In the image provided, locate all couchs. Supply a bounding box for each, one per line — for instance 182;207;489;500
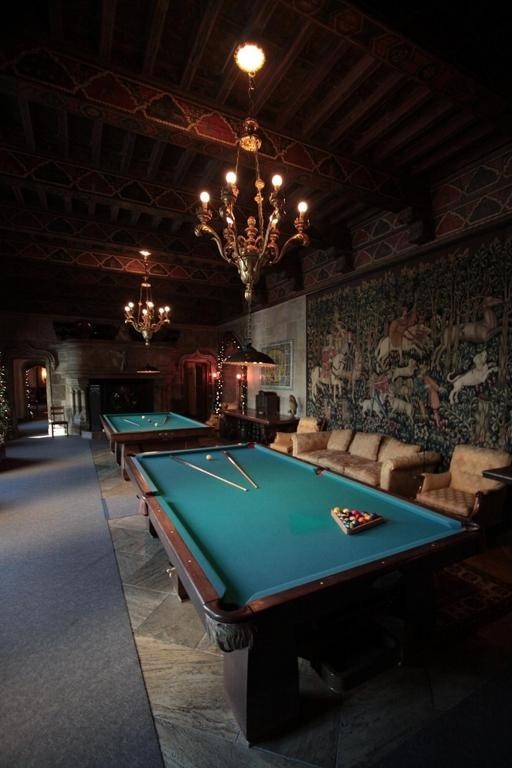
292;431;443;496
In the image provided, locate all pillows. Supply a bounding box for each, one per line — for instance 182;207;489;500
350;431;381;460
378;435;421;463
327;429;355;452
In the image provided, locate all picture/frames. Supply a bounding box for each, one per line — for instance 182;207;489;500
259;340;293;390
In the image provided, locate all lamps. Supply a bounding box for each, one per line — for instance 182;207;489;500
236;373;244;380
195;41;311;301
41;366;46;383
123;251;171;347
223;301;277;367
212;372;220;379
136;363;161;375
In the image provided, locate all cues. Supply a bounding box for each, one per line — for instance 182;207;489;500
175;456;247;492
123;419;140;427
163;415;168;424
223;449;259;490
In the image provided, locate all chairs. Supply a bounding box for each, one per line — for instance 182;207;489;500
413;445;512;551
270;416;325;454
206;403;239;440
50;406;68;438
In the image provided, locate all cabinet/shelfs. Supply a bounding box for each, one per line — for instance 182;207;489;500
224;408;300;446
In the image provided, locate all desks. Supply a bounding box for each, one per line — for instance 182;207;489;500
124;442;483;748
100;411;217;480
482;465;512;485
